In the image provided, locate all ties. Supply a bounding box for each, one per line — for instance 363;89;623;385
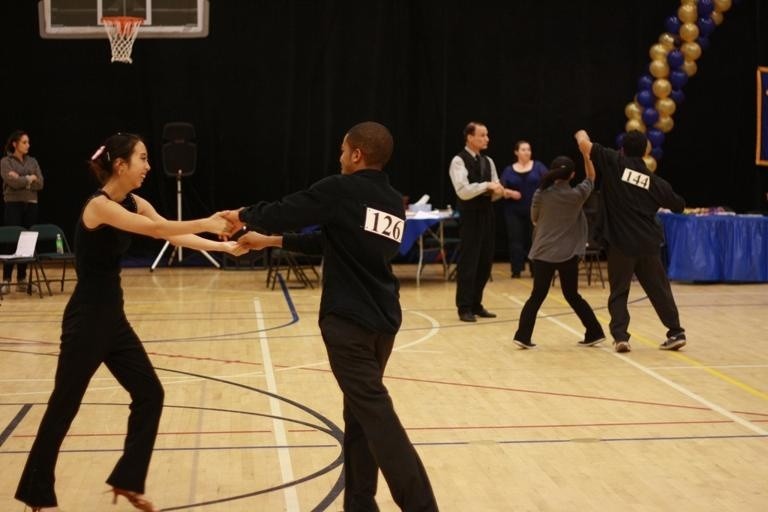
476;154;485;178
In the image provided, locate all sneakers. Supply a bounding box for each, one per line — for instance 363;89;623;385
660;335;686;350
578;337;606;346
1;281;10;295
16;279;37;293
513;340;537;349
616;341;632;352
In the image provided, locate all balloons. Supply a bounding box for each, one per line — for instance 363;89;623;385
616;1;733;173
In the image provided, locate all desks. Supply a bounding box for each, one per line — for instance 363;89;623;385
398;210;459;288
660;213;767;282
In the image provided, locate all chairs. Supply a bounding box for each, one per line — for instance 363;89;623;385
552;233;606;290
32;223;79;298
0;226;43;297
265;236;321;289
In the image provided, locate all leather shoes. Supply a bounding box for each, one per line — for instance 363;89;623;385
461;311;475;322
477;310;496;317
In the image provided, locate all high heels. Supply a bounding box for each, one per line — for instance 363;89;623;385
113;487;160;512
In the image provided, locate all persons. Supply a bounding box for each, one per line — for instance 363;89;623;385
575;127;687;351
512;139;607;350
0;132;45;295
499;141;552;280
218;120;441;512
13;132;252;511
448;120;512;322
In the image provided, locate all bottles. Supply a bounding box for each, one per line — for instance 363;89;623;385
55;233;64;255
